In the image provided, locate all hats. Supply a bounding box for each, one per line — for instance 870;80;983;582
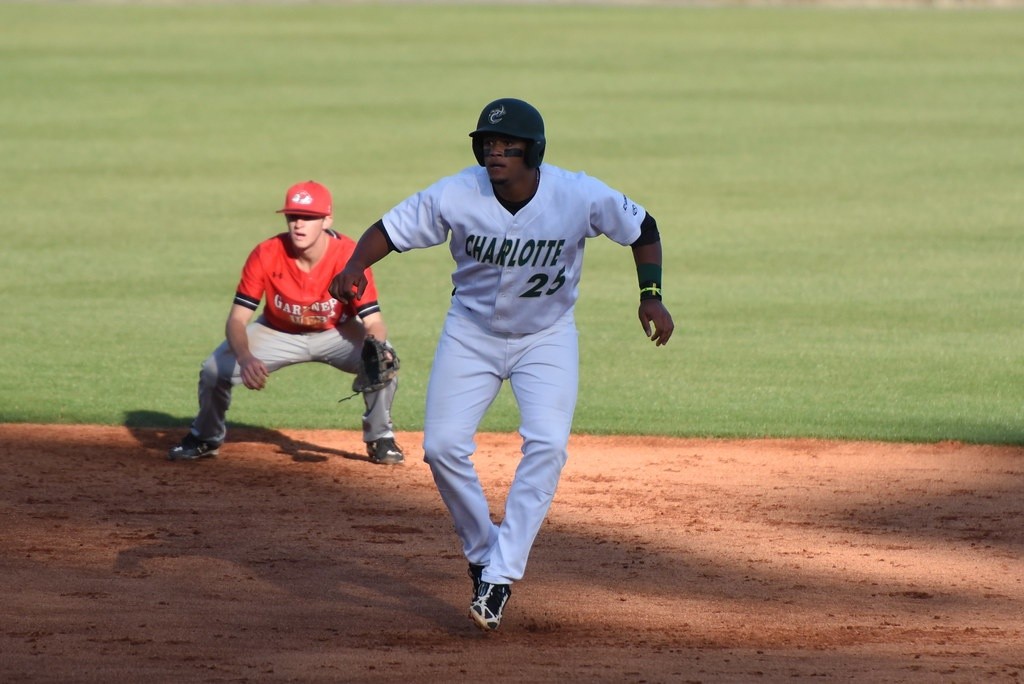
276;180;331;216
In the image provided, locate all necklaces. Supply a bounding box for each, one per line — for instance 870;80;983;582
535;169;540;184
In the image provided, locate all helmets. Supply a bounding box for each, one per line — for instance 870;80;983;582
469;98;546;167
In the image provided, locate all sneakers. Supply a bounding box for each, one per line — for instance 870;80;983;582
366;438;404;463
470;582;512;631
468;562;488;633
170;432;220;459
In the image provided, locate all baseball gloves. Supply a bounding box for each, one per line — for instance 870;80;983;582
362;332;400;392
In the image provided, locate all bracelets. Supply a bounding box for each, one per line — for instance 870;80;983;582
640;283;662;297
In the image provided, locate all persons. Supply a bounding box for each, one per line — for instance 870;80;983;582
167;177;407;465
328;97;675;630
